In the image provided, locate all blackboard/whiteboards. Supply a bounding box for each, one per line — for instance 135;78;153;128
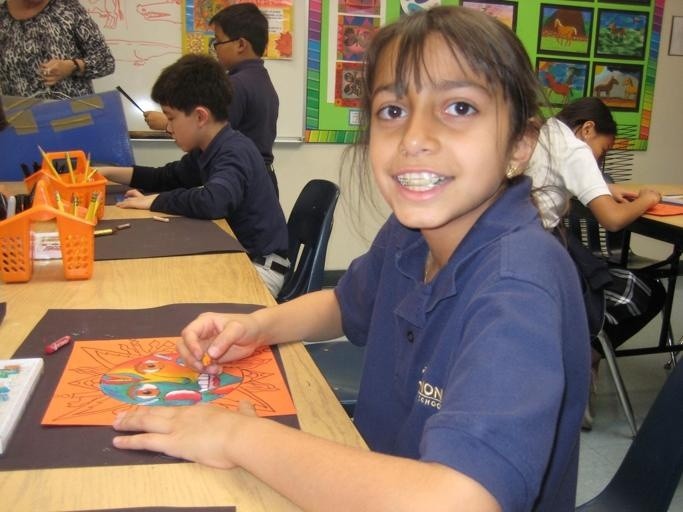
78;0;308;144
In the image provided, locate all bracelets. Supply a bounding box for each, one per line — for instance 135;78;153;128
72;58;80;72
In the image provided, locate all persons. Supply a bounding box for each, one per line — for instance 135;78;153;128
0;0;116;101
143;3;280;201
520;94;665;432
85;53;290;300
110;7;591;510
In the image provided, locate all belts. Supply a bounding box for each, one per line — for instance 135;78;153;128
250;255;288;274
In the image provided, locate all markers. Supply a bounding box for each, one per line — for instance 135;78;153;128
45;336;70;354
95;228;116;236
118;224;131;231
153;216;170;223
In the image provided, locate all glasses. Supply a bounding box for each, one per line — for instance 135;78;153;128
213;38;240;52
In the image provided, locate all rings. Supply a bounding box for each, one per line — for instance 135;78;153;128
40;70;49;75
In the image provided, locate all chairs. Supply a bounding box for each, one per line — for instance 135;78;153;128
566;172;678;368
575;348;683;512
276;178;339;302
307;341;366;418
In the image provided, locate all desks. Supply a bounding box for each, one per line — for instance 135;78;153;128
610;181;683;359
0;184;400;512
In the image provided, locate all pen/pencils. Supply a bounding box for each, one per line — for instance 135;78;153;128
0;192;31;220
55;189;100;222
37;144;98;184
201;351;212;368
21;159;41;178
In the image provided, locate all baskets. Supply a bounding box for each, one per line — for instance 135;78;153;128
26;151;108;219
1;181;96;283
